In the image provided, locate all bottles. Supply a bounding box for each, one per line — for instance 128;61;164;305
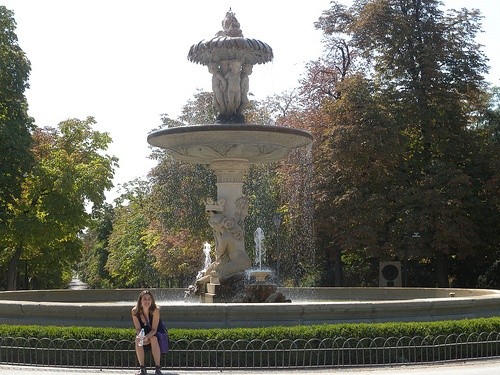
138;328;145;346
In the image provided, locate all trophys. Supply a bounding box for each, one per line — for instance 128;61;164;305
208;214;252;283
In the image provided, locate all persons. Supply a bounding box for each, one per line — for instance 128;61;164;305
220;60;241;114
208;63;227;114
131;290;168;375
240;63;253;114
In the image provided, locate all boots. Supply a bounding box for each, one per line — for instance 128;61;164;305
154;365;163;375
135;365;147;375
